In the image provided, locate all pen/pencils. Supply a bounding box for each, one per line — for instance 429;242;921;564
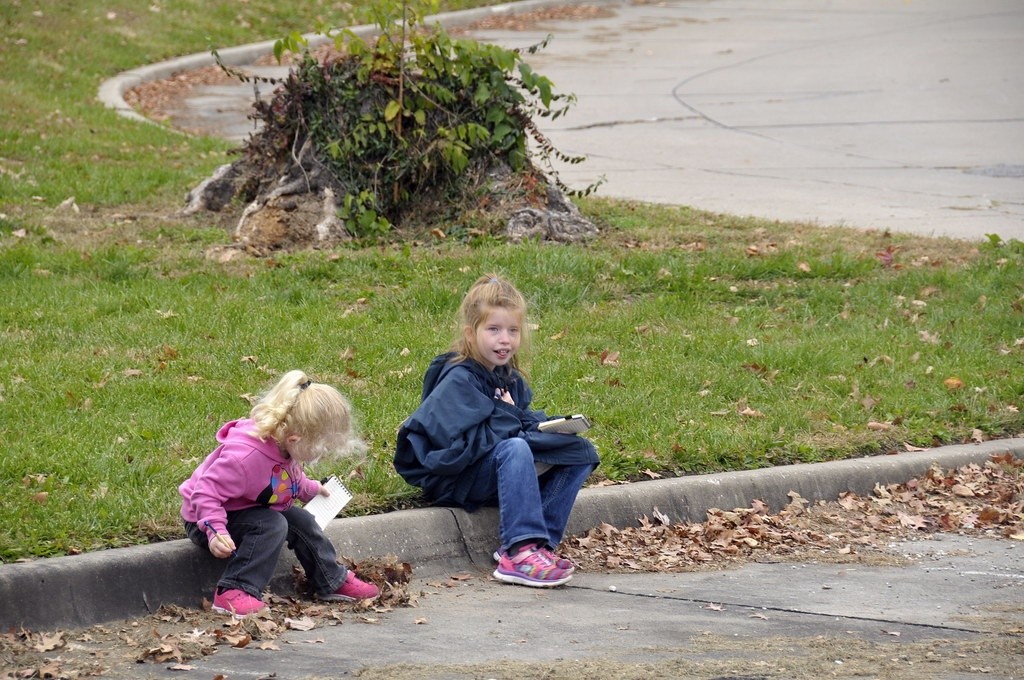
495;393;503;400
204;520;239;558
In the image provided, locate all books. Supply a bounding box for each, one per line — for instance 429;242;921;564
300;473;354;532
537;413;592;434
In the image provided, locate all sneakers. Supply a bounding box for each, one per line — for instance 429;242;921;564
211;587;271;619
493;544;572;587
314;570;380;602
493;545;574;574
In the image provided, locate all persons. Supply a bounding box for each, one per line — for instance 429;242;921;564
392;272;601;588
178;370;380;621
